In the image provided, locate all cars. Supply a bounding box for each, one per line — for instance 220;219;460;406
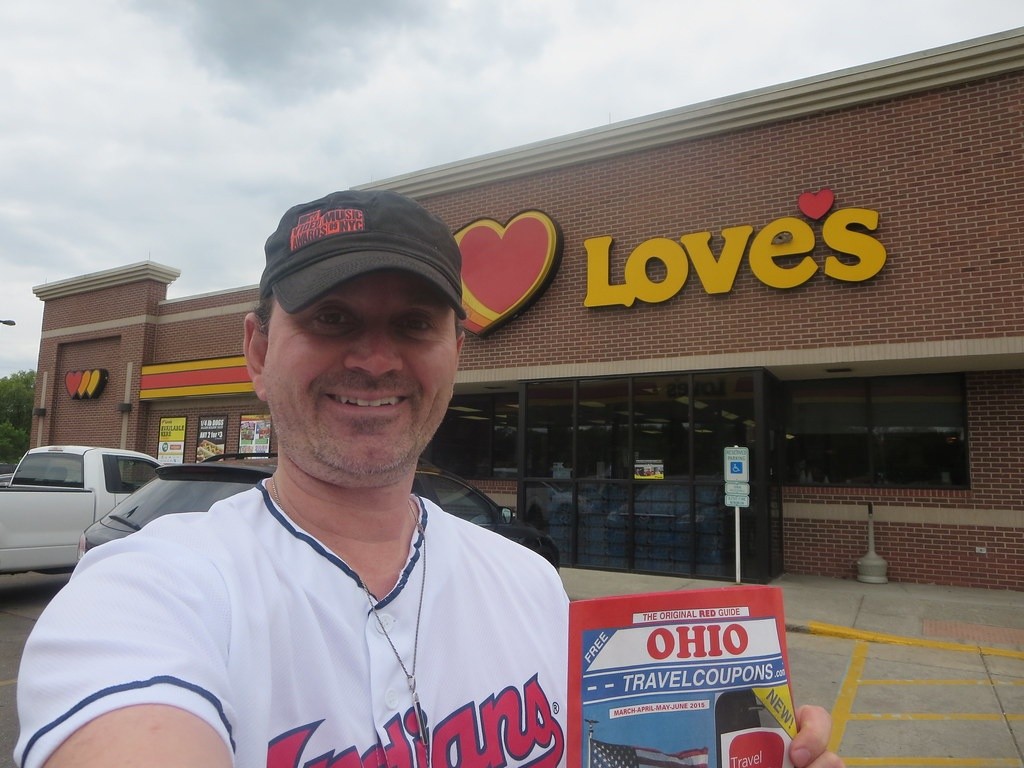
604;477;760;565
493;468;592;527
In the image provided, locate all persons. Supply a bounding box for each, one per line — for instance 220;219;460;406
15;191;845;768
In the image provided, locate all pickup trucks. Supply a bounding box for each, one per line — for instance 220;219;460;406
0;446;165;575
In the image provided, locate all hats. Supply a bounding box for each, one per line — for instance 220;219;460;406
258;189;467;320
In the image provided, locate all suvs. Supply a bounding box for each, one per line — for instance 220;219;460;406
78;453;559;573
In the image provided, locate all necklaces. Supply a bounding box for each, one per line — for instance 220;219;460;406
267;475;431;745
